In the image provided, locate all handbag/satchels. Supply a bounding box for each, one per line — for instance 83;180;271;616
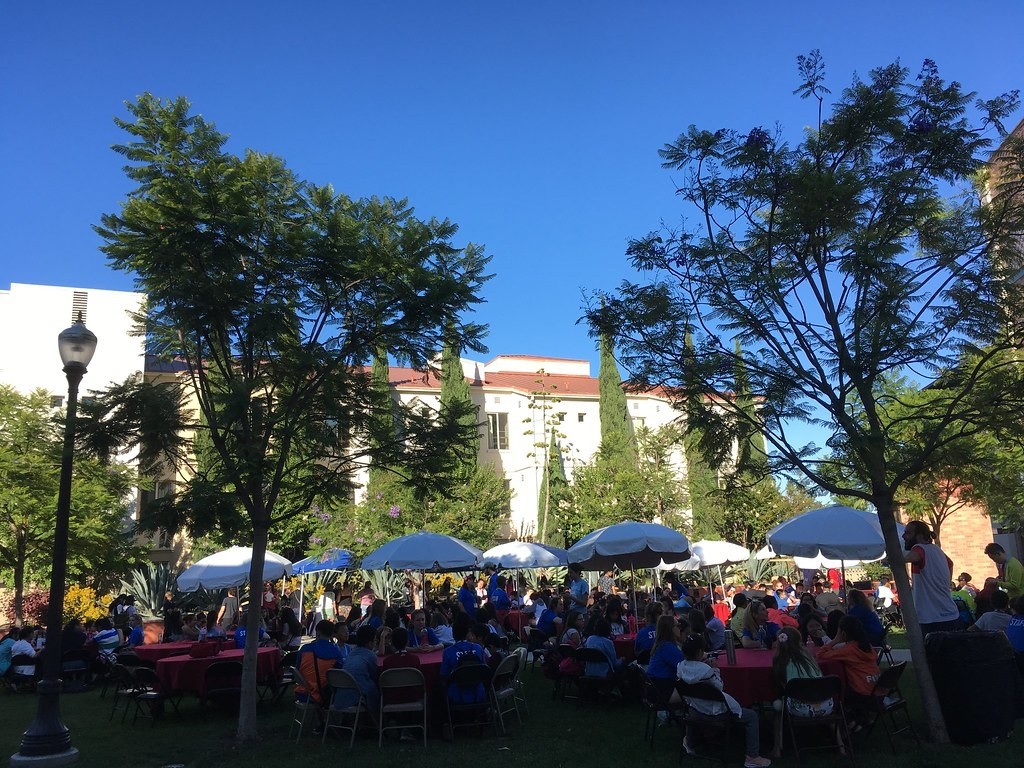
320;685;334;710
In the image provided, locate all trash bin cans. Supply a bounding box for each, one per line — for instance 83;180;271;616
924;630;1018;747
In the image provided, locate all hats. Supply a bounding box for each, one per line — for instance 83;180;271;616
464;576;473;582
482;562;495;570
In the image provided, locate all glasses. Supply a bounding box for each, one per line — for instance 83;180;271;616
577;618;585;622
673;623;681;629
751;600;758;607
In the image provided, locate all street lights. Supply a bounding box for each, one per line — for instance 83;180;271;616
6;306;99;768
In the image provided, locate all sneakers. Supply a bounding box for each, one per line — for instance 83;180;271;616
744;755;771;768
683;736;695;756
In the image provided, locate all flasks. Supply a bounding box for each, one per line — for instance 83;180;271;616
724;630;737;665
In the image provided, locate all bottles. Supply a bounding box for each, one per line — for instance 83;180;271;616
198;627;207;641
629;617;636;635
758;625;766;648
421;627;428;647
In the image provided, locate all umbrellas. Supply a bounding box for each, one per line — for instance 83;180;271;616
754;502;912;616
176;521;750;646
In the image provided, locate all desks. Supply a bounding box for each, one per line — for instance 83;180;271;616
152;646;283;693
710;604;731;628
611;635;636;659
703;646;846;701
133;639;236;662
509;610;528;641
377;649;444;673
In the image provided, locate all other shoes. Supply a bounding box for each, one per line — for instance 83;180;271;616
841;715;874;739
11;684;21;694
443;727;451;742
400;729;416;741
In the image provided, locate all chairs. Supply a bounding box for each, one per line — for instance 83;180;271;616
10;598;975;766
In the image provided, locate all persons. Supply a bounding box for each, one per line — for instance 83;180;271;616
881;521;1024;655
291;562;898;768
0;594;144;694
163;581;309;651
